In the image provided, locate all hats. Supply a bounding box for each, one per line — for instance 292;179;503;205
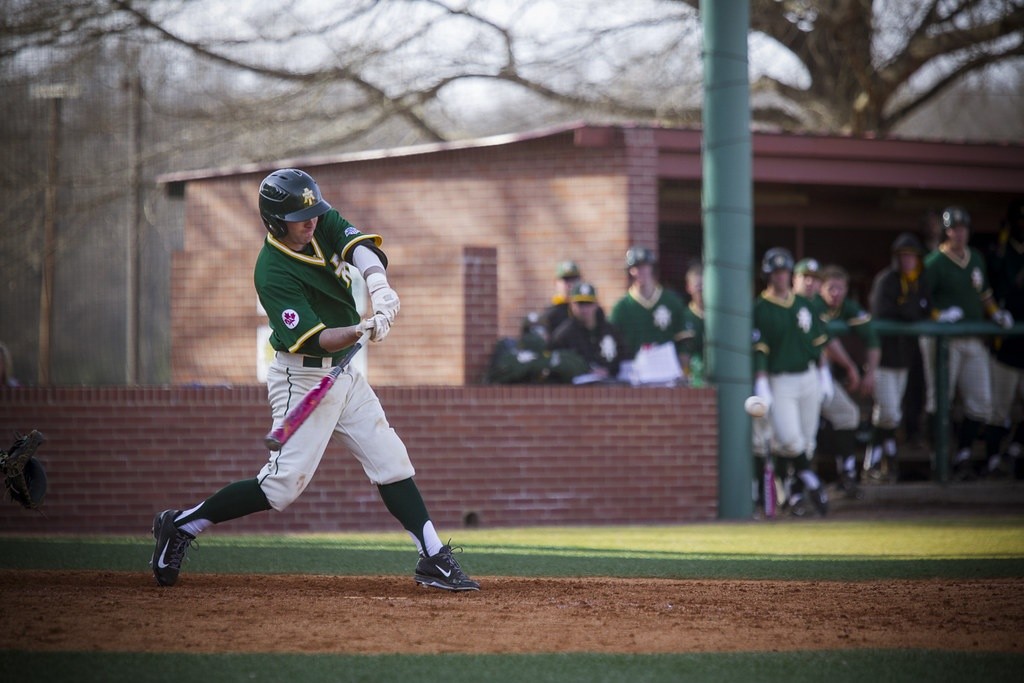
556;262;580;277
762;248;794;273
626;246;653;269
794;257;822;277
523;314;551;331
894;233;922;255
571;283;597;302
822;264;847;277
943;205;971;226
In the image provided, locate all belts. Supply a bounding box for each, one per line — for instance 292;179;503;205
302;356;343;367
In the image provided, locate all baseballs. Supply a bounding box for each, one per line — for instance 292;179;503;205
743;395;769;418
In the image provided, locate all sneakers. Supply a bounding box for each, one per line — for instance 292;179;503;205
149;508;199;586
415;538;482;590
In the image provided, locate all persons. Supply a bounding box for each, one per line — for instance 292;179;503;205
488;207;1024;518
150;169;481;591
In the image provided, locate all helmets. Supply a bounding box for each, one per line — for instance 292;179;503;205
258;169;331;238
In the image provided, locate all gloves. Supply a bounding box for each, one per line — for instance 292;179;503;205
993;308;1014;328
371;287;401;327
356;314;390;342
937;306;964;323
820;369;834;404
756;377;772;417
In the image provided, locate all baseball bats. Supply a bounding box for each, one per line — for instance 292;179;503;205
260;329;374;452
